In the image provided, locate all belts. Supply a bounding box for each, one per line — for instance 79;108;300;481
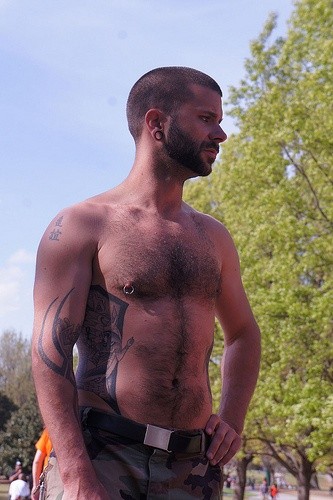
76;406;213;456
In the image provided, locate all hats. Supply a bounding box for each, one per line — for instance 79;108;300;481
15;461;21;465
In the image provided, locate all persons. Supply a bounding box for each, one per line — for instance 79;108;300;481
30;66;262;500
221;471;279;500
7;427;56;499
